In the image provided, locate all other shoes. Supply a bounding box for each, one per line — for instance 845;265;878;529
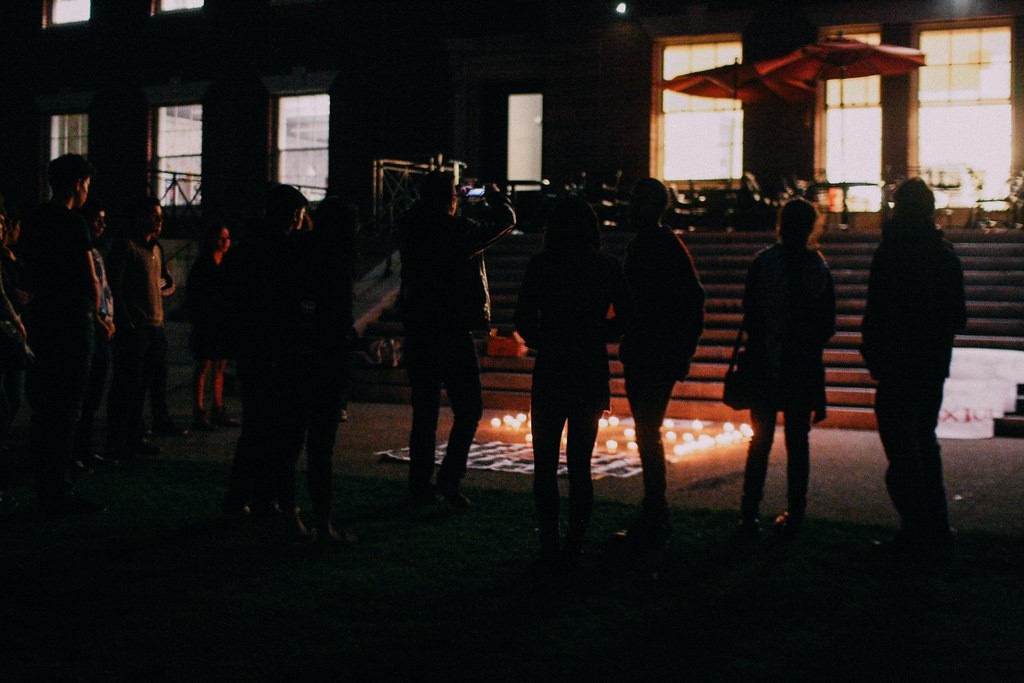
286;507;337;541
405;484;471;509
79;415;189;464
30;490;107;517
876;530;952;564
614;497;670;552
733;524;800;556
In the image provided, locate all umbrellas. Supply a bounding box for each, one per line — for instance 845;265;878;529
654;33;926;181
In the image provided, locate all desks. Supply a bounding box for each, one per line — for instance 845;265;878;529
812;182;877;226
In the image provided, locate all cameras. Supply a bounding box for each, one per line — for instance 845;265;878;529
467;186;487;196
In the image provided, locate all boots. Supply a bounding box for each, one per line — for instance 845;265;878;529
191;399;241;432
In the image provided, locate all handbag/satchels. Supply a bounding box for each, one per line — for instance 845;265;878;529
723;361;757;408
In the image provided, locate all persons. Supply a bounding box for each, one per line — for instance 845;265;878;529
0;154;189;515
859;177;967;560
727;173;760;231
613;177;707;545
185;185;396;546
398;173;515;512
516;196;622;560
736;199;836;537
